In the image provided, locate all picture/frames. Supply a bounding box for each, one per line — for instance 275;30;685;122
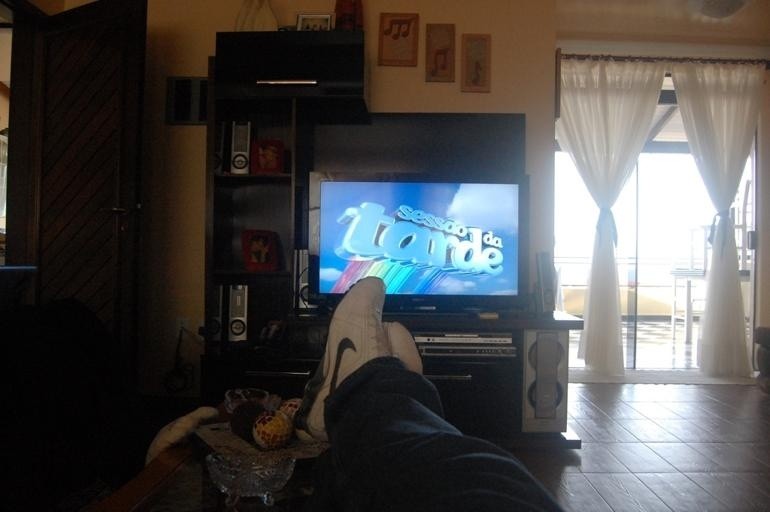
296;14;333;32
377;11;455;81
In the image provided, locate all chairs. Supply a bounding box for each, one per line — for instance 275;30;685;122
670;224;749;344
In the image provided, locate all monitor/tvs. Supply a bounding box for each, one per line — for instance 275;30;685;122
308;171;530;316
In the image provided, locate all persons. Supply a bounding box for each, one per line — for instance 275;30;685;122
293;276;566;512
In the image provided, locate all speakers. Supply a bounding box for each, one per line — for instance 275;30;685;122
298;250;308;310
230;120;251;175
228;284;248;342
521;329;569;433
535;251;555;312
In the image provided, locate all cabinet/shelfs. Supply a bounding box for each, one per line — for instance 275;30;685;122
202;30;585;453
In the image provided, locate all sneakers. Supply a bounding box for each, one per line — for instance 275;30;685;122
294;276;424;445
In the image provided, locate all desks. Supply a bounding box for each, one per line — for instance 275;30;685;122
82;394;316;511
